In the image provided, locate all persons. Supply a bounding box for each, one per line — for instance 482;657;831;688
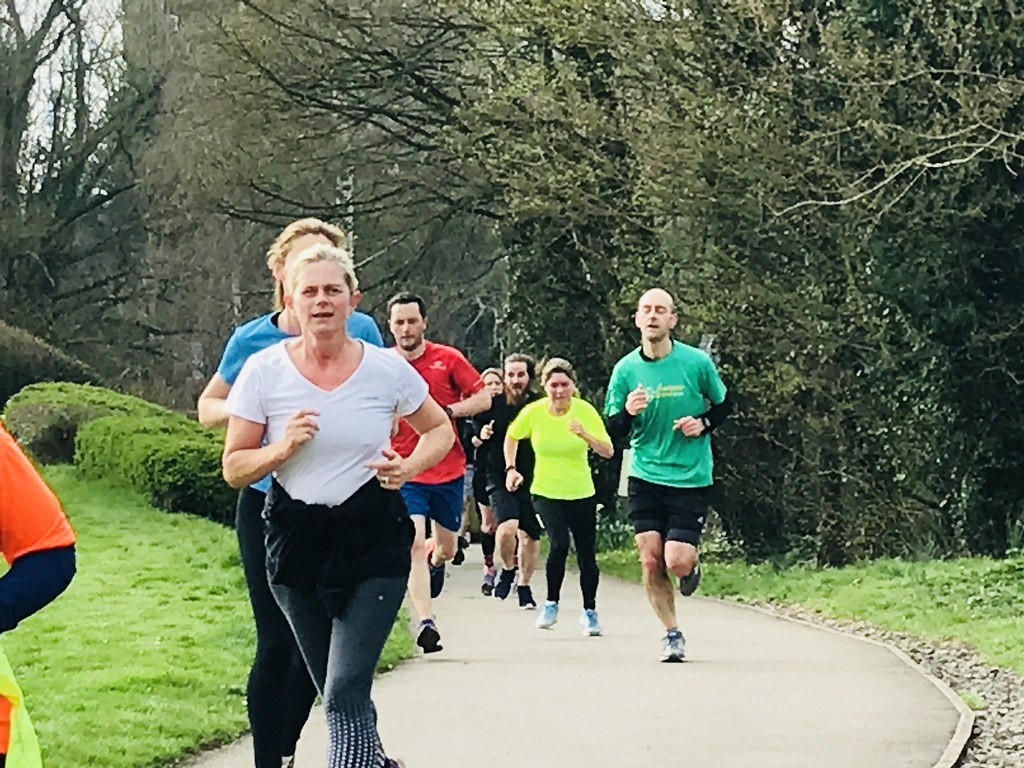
197;216;385;768
425;368;519;598
387;292;491;654
504;358;614;637
471;353;541;610
604;286;732;662
222;246;458;768
0;425;78;768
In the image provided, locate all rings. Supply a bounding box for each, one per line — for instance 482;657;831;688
382;477;389;485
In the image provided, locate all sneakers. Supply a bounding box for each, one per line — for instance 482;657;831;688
660;630;685;662
417;623;442;653
680;563;700;596
517;585;536;609
428;551;446;598
494;566;519;601
535;600;558;628
583;609;600;636
482;564;497;597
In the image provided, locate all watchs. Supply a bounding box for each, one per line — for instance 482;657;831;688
696;414;711;436
442;405;453;420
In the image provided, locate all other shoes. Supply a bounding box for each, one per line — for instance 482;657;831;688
451;534;469;564
382;756;406;768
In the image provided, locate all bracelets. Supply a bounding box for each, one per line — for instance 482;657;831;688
476;431;484;442
505;466;516;472
